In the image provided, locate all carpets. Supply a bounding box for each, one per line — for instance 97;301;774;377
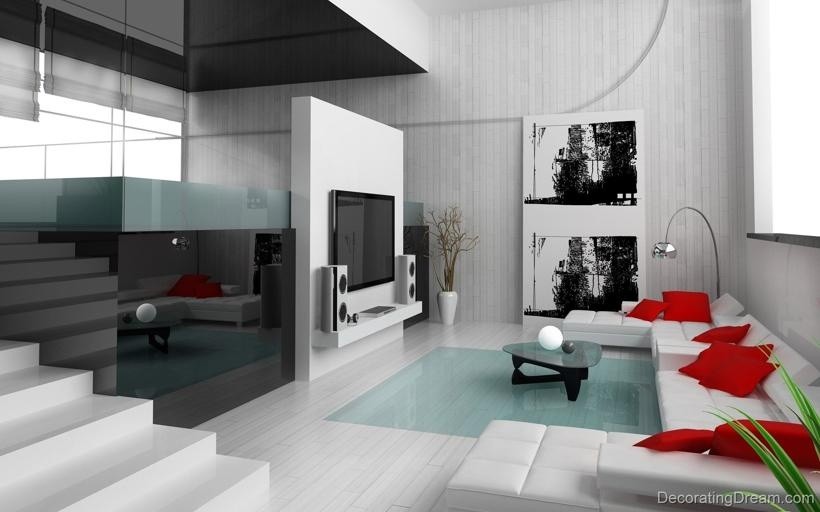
323;345;665;438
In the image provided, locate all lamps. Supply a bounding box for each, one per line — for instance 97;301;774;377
652;207;721;299
167;232;191;249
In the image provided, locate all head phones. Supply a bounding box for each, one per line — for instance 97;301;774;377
347;313;359;323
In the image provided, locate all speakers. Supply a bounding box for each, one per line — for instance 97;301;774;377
321;265;348;331
396;254;416;304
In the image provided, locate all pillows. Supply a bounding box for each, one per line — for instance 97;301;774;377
626;290;819;469
167;273;223;298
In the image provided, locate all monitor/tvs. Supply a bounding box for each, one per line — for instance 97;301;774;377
331;190;395;292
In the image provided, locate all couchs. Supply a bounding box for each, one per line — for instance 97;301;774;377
118;275;261;330
451;293;819;512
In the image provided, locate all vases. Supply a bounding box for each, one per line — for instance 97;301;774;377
438;291;457;325
